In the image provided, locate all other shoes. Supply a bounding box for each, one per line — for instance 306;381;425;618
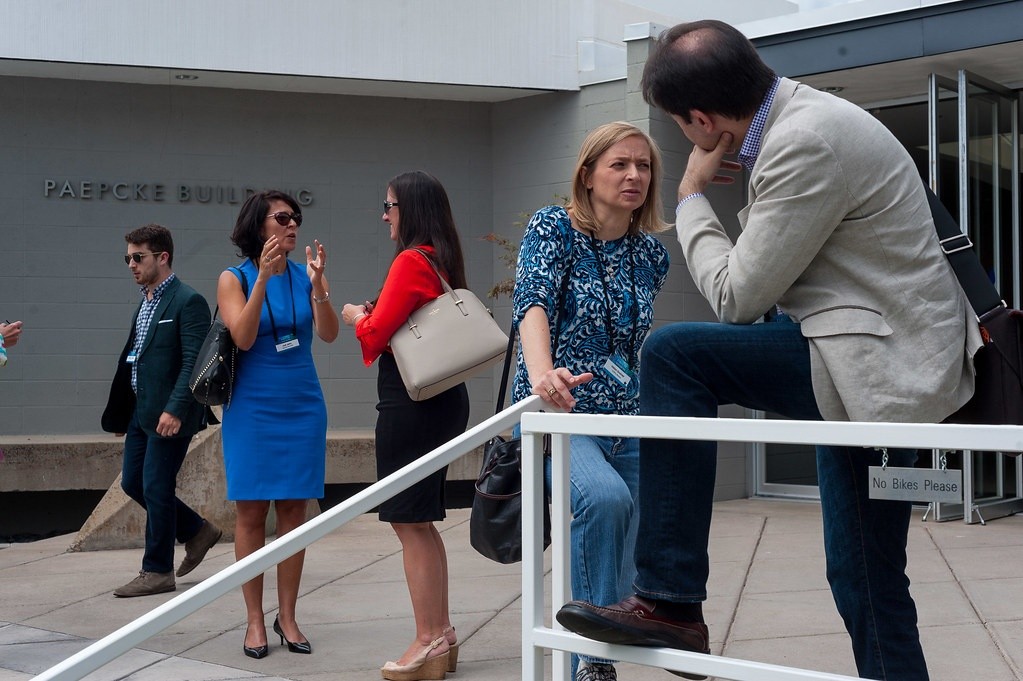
574;659;618;681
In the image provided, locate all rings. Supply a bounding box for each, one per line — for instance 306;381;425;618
549;387;556;397
265;256;270;260
321;263;326;267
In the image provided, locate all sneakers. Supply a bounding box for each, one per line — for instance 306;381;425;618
113;567;176;596
176;518;222;577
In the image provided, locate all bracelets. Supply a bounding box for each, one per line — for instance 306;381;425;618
314;292;329;304
353;313;365;328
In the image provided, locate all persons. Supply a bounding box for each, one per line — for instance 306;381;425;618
0;320;23;368
512;121;676;681
342;170;470;681
555;20;985;681
100;224;223;597
217;190;339;659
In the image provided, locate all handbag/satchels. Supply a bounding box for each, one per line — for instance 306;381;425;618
470;436;551;563
188;265;249;407
936;309;1023;429
389;251;517;402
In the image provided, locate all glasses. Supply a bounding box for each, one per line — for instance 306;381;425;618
124;251;165;265
383;199;398;215
265;211;303;229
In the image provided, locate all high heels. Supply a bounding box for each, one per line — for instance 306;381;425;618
244;624;268;659
443;625;459;671
382;635;450;681
274;613;311;654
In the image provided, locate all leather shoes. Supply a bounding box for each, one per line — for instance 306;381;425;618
556;590;710;679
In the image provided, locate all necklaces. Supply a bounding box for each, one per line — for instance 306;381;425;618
597;232;617;274
